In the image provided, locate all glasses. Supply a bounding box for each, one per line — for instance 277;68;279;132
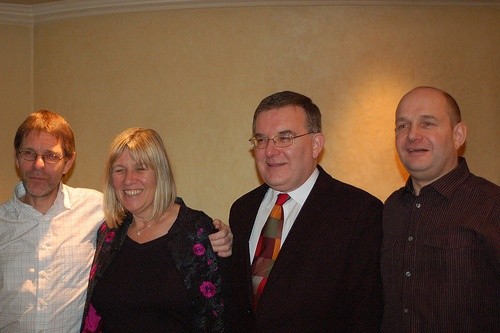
248;131;313;149
18;150;65;165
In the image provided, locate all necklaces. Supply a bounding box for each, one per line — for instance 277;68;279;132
132;222;152;235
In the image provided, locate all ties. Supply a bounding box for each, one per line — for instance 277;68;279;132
251;193;291;318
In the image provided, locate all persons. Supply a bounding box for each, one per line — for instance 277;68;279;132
80;128;226;333
229;90;384;333
0;109;233;333
379;86;500;333
